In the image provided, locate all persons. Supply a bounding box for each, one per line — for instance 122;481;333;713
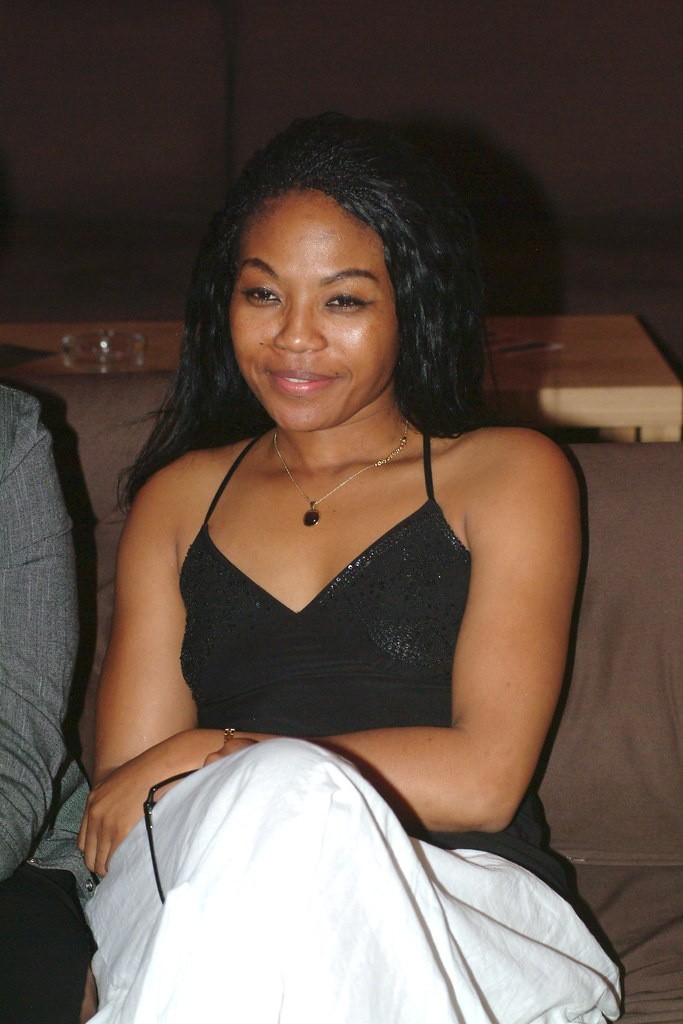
0;383;99;1024
77;112;622;1024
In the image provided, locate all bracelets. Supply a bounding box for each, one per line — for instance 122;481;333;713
224;728;235;745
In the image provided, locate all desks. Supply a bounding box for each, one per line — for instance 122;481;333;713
1;313;683;447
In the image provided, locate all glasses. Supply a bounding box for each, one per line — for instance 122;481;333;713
141;771;195;902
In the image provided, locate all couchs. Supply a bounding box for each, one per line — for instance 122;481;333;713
1;371;683;1023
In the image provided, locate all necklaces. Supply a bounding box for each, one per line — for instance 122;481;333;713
274;419;409;527
